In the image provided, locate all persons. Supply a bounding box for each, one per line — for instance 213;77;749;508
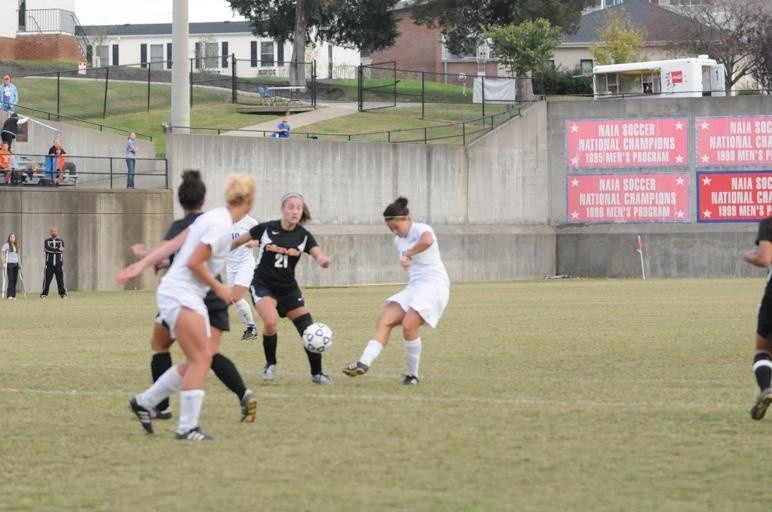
125;131;138;189
132;167;258;424
742;214;771;420
343;196;450;389
41;226;69;300
0;74;19;112
232;191;335;386
270;118;290;138
0;114;71;186
226;213;261;340
0;232;21;300
117;173;255;440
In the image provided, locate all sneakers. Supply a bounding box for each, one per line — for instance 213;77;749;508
752;388;772;419
130;329;418;439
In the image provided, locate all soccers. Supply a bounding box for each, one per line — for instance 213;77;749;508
302;323;334;352
